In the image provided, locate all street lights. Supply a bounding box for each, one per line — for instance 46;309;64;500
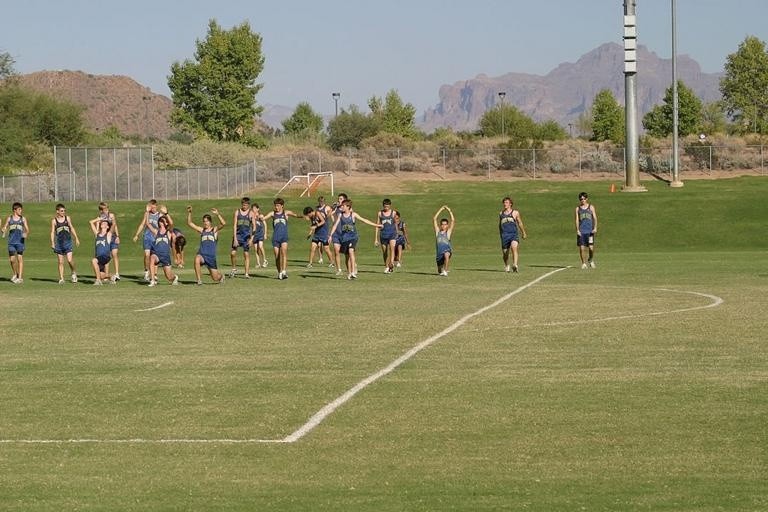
568;123;573;138
332;92;341;115
497;92;506;143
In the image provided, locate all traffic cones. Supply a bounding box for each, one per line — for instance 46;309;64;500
608;183;617;193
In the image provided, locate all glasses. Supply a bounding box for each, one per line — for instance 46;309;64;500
580;198;587;200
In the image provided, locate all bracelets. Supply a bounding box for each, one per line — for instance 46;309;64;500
447;209;452;213
251;231;256;236
116;236;119;238
164;212;168;217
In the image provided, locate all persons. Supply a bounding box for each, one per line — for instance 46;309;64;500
250;203;270;270
89;216;116;286
185;205;226;286
392;209;410;269
50;203;80;284
143;203;178;288
574;192;598;270
373;199;398;275
132;199;165;282
96;201;121;284
432;204;455;277
1;202;30;284
228;196;256;279
303;194;384;279
167;229;188;269
257;198;310;281
497;197;527;274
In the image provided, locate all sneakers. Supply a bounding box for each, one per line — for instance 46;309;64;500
58;278;64;285
245;272;252;278
144;271;158;286
220;274;226;283
195;280;202;286
255;260;268;268
10;275;23;284
347;270;358;280
384;260;402;273
172;275;179;285
306;259;343;277
440;270;448;276
229;269;238;277
581;261;595;269
71;273;78;283
92;272;120;287
278;271;288;279
504;265;518;272
